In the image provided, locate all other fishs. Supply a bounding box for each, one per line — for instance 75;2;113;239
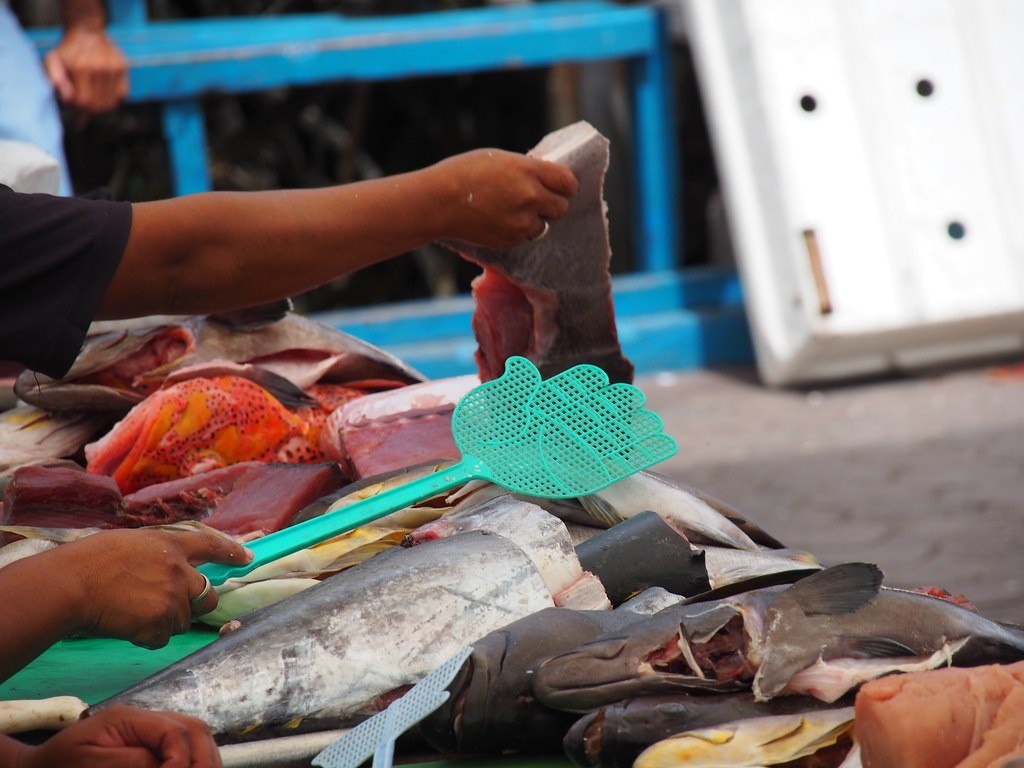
453;122;633;382
437;463;788;553
74;486;1016;768
0;299;433;473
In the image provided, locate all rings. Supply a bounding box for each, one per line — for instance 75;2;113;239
528;220;553;243
189;572;214;606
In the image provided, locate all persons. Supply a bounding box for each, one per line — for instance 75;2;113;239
0;0;128;196
0;146;581;380
0;528;253;767
